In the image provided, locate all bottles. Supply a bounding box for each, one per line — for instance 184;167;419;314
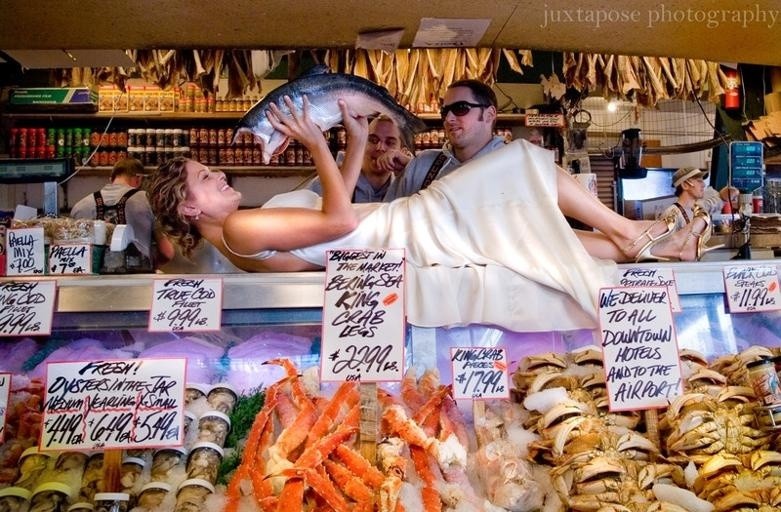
126;128;134;147
35;128;45;146
145;128;155;147
8;146;89;165
57;127;65;146
82;127;89;146
199;127;207;147
47;128;54;145
189;128;198;146
126;147;190;166
225;128;232;146
90;152;99;166
155;128;164;147
65;127;72;147
270;147;313;165
218;148;226;166
117;128;128;147
252;147;261;165
18;128;26;146
226;147;234;166
108;150;116;166
243;148;252;166
191;147;197;161
172;129;182;148
117;150;125;161
99;150;108;166
174;84;257;112
234;129;260;147
135;128;144;147
164;128;172;147
198;147;208;165
217;128;225;147
100;128;108;146
8;128;17;146
234;148;243;166
27;128;35;146
208;128;217;148
182;129;189;147
90;128;100;146
72;128;82;148
208;148;217;166
109;128;117;146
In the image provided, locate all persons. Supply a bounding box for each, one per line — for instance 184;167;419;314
143;94;729;334
381;80;508;203
659;166;740;228
70;157;176;274
303;114;414;203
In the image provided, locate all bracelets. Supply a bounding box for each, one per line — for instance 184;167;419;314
405;149;412;158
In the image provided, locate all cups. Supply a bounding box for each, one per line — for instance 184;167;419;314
753;195;763;213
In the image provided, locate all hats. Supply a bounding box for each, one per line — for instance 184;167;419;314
672;167;709;188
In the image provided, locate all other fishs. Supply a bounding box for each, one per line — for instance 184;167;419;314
229;64;431;163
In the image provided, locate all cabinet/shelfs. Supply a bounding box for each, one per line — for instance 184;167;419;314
1;85;564;179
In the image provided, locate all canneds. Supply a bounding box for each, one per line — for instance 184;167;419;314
9;124;128;167
190;129;263;166
270;129;348;167
746;359;781;424
411;128;514;156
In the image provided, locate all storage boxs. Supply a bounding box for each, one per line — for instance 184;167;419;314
0;154;77;184
488;82;544;112
9;88;99;113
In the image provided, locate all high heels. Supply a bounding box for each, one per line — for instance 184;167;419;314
625;208;678;263
680;205;724;262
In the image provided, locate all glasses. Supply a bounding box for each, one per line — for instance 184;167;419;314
441;101;486;116
